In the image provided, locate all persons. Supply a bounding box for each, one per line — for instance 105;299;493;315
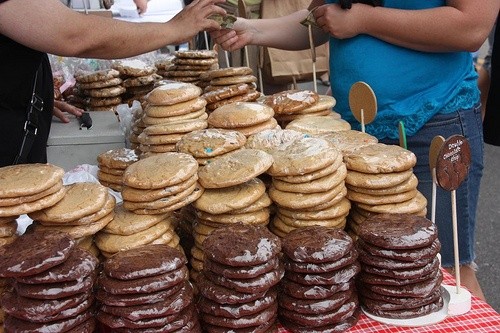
209;0;499;304
60;0;294;93
0;0;226;165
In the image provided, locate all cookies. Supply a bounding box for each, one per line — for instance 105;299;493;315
0;49;443;333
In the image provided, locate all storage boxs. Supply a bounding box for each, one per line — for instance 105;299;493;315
46;110;125;174
